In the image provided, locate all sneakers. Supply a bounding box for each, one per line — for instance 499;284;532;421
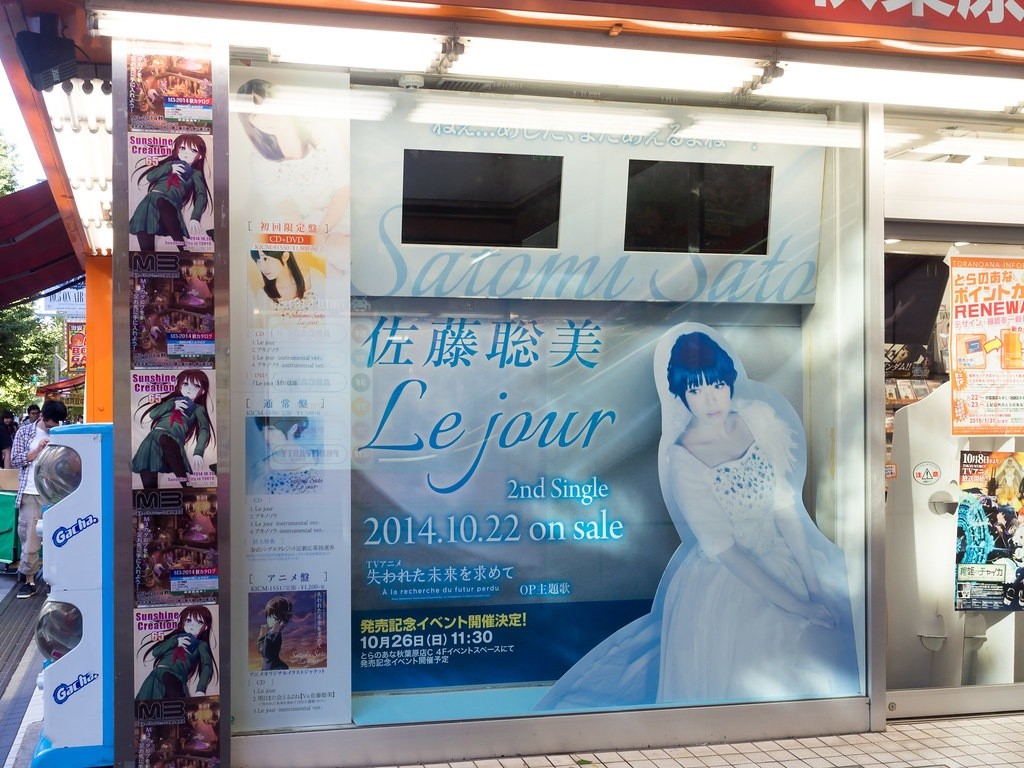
17;582;36;599
46;583;51;596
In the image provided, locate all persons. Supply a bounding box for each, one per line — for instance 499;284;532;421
0;403;84;470
896;343;924;363
250;249;326;315
252;417;316;472
556;321;863;707
10;400;68;597
233;80;350;243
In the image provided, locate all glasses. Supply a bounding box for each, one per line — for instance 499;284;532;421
44;419;52;430
30;413;39;416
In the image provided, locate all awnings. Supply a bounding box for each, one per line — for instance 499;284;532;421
0;179;88;313
35;375;85;397
